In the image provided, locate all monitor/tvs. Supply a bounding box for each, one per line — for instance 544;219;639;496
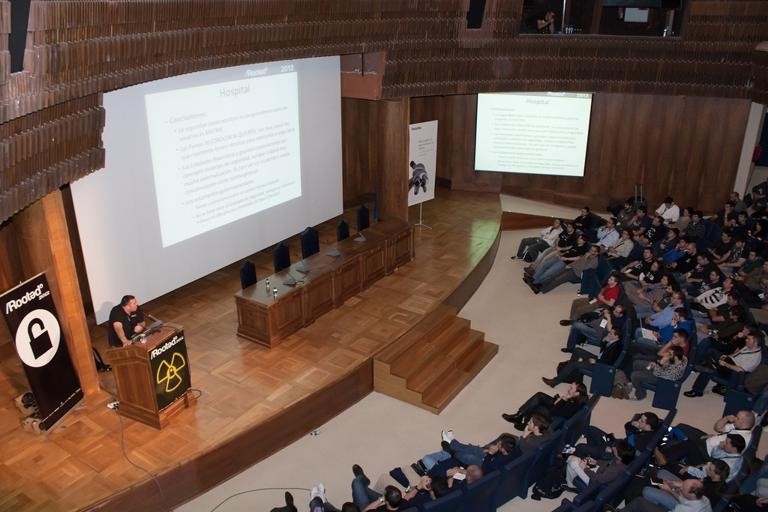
132;328;151;342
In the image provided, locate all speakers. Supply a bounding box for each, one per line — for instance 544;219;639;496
8;0;31;73
466;0;486;29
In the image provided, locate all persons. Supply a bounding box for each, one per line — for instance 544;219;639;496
512;181;768;400
271;427;522;512
107;295;149;349
536;7;556;35
499;382;757;512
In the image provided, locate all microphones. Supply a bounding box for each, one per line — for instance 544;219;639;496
283;270;297;286
295;254;311;274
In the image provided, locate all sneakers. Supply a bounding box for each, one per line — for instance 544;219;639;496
311;483;326;503
511;256;522;259
631;396;638;401
441;430;453;445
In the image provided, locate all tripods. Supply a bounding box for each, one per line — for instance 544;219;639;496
414;203;432;230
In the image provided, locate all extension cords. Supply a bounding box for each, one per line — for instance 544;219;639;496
107;401;120;409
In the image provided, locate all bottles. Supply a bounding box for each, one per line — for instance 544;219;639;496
265;278;272;290
273;287;278;299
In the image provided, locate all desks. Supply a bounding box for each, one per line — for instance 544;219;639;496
233;214;418;351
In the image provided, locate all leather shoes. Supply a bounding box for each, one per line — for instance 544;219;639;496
352;464;370;485
523;266;541;294
503;413;525;432
684;390;703;397
562;348;572;353
694;364;714;373
560;320;572;326
285;492;298;512
542;377;554;387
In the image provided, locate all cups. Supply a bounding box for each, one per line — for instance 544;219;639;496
140;333;147;344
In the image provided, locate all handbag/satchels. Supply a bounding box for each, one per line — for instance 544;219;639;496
522;248;538;262
613;369;631;400
532;472;567;500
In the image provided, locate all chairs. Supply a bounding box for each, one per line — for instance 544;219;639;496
237;258;261;290
355;205;372;231
510;190;768;413
298;225;322;259
270;391;767;509
336;218;350;239
270;240;293;273
